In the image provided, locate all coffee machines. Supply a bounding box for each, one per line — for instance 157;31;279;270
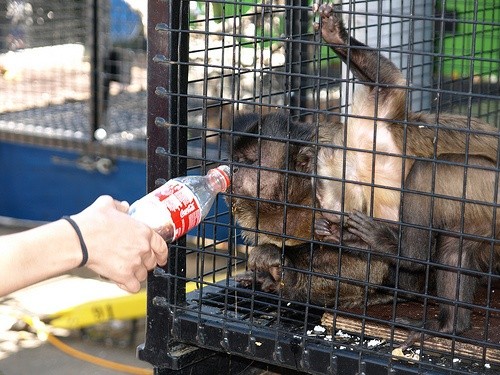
127;164;235;244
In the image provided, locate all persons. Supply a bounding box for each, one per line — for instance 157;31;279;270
1;194;168;296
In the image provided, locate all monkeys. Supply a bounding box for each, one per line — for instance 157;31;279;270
225;4;500;351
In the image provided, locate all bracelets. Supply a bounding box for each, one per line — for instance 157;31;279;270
60;214;88;268
